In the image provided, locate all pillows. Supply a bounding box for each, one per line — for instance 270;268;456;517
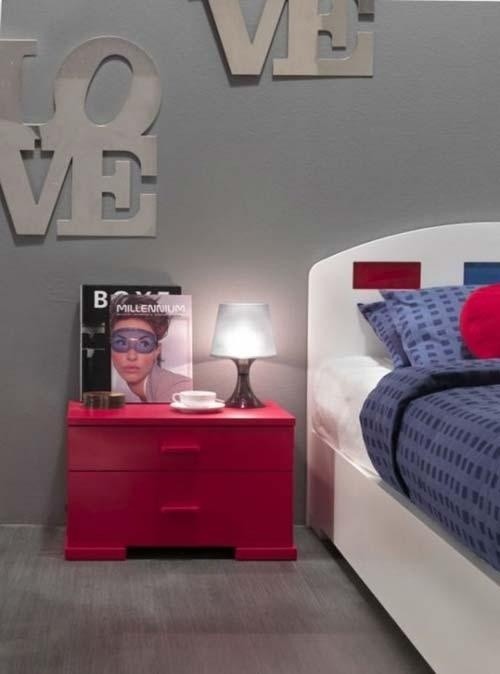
464;288;500;359
353;287;405;373
382;286;500;361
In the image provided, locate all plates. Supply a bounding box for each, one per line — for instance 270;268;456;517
169;401;226;414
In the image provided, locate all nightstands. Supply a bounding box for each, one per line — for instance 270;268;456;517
63;395;301;564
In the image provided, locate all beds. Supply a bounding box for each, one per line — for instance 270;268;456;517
303;220;500;673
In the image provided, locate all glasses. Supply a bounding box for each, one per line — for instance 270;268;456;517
109;326;158;353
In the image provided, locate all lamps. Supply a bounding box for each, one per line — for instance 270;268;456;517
210;302;283;408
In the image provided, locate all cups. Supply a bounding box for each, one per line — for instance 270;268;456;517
172;389;217;407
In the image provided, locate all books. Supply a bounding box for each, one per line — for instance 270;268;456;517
79;285;183;401
